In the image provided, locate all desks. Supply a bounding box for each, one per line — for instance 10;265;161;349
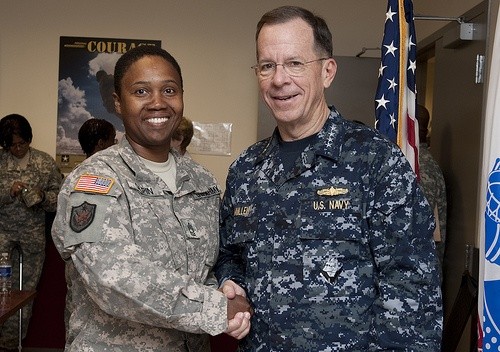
0;288;39;327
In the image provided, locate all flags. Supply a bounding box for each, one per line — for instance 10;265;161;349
477;0;500;352
373;0;420;180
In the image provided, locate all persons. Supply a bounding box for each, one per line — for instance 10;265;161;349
413;104;446;285
77;118;116;159
50;45;250;352
214;5;443;352
170;117;194;158
0;114;64;352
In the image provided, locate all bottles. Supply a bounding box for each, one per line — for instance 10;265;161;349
0;252;12;297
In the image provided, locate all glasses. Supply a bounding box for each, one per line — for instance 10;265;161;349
250;57;331;76
105;138;118;144
